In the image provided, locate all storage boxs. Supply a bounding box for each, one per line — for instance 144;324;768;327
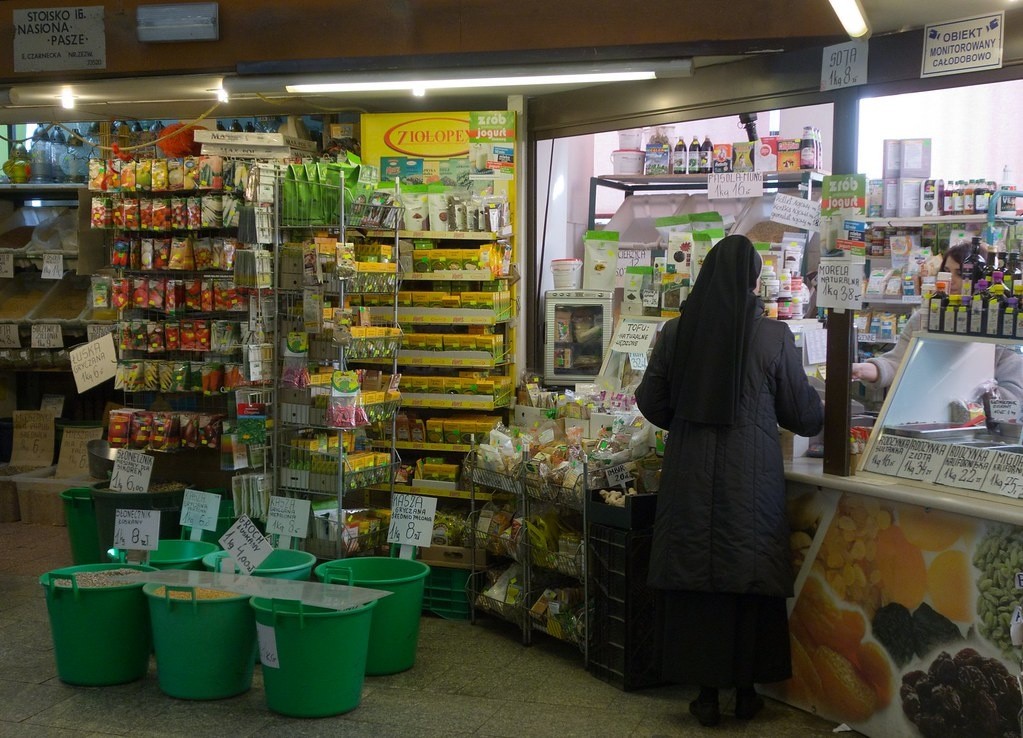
0;201;108;529
284;241;510;619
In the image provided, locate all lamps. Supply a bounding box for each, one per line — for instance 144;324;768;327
221;58;695;95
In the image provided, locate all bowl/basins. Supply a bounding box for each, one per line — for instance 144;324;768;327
86;439;171;479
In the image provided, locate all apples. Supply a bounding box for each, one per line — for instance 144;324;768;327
165;326;208;344
135;172;152;186
112;278;176;310
121;173;135;188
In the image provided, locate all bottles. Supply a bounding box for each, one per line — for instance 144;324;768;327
3;119;256;185
849;425;872;476
942;178;997;216
674;136;687;174
555;306;602;368
920;238;1023;340
688;135;701;174
760;263;805;320
996;164;1017;217
701;135;714;172
558;530;584;578
800;125;822;170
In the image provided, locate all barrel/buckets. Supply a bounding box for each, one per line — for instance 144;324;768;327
549;258;584;290
609;150;646;175
618;128;643;151
36;478;432;719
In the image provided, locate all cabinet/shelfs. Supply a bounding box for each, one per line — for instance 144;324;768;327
523;454;590;674
854;211;1023;363
316;232;519;624
0;182;96;371
587;173;823;231
272;168;403;557
463;450;526;645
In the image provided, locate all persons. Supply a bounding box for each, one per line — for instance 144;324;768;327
851;245;1023;424
633;234;825;727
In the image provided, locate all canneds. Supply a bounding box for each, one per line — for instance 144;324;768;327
864;226;897;257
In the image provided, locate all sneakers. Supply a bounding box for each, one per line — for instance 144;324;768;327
689;698;719;725
736;695;765;721
